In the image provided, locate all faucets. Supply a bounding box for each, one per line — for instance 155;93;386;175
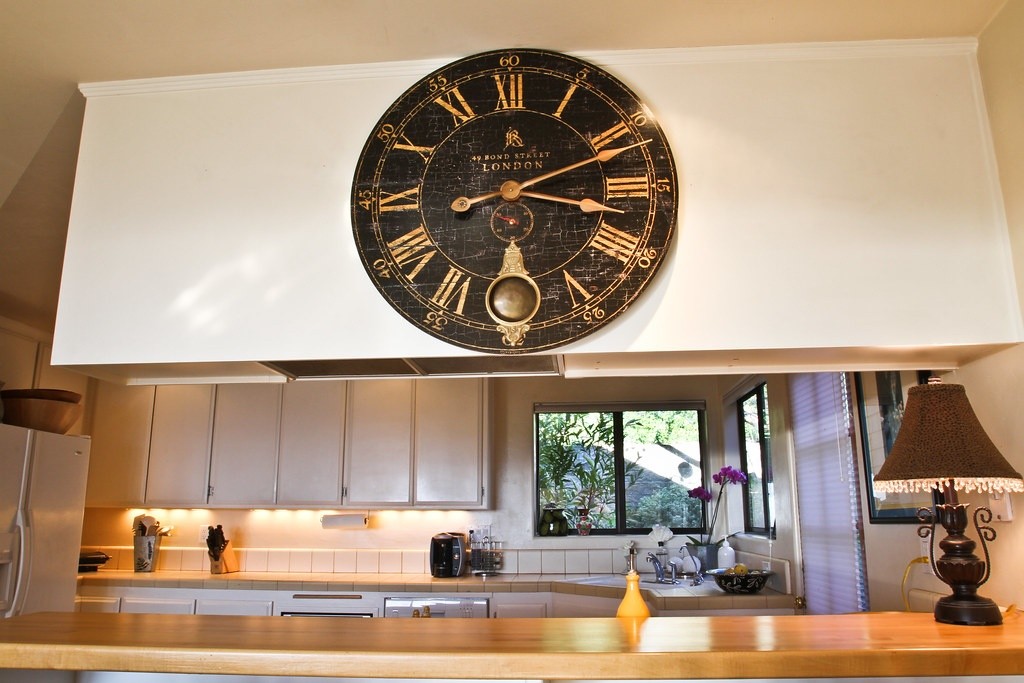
641;551;679;585
679;546;704;586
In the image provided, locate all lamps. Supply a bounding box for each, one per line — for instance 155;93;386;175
864;376;1024;628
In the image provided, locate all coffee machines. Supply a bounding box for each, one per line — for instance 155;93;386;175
430;531;466;578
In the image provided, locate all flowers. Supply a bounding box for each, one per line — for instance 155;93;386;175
684;465;749;545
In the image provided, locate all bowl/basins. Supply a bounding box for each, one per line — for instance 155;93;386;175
705;569;775;594
1;389;83;435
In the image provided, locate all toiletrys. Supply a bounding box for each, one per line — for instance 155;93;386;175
717;535;735;569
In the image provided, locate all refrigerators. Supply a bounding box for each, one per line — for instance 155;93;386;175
0;424;92;683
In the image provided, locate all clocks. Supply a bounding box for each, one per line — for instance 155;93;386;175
350;46;680;356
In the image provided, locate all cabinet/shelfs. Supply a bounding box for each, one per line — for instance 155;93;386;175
76;583;279;614
206;380;342;511
344;379;493;511
491;592;554;621
88;384;215;514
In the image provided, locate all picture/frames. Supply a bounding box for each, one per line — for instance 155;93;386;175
852;370;963;526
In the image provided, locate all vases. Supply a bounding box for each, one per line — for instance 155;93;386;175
686;544;720;573
538;506;568;536
576;508;592;535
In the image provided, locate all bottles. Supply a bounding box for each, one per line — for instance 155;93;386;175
616;571;650;617
539;507;569;536
655;543;668;571
575;509;592;535
625;549;638;571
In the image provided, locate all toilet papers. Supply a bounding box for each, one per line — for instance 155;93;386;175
321;513;368;529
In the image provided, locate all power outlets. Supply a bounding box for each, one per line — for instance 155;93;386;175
761;561;771;588
199;525;209;543
918;533;936;576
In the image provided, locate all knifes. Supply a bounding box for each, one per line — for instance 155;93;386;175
206;525;226;558
